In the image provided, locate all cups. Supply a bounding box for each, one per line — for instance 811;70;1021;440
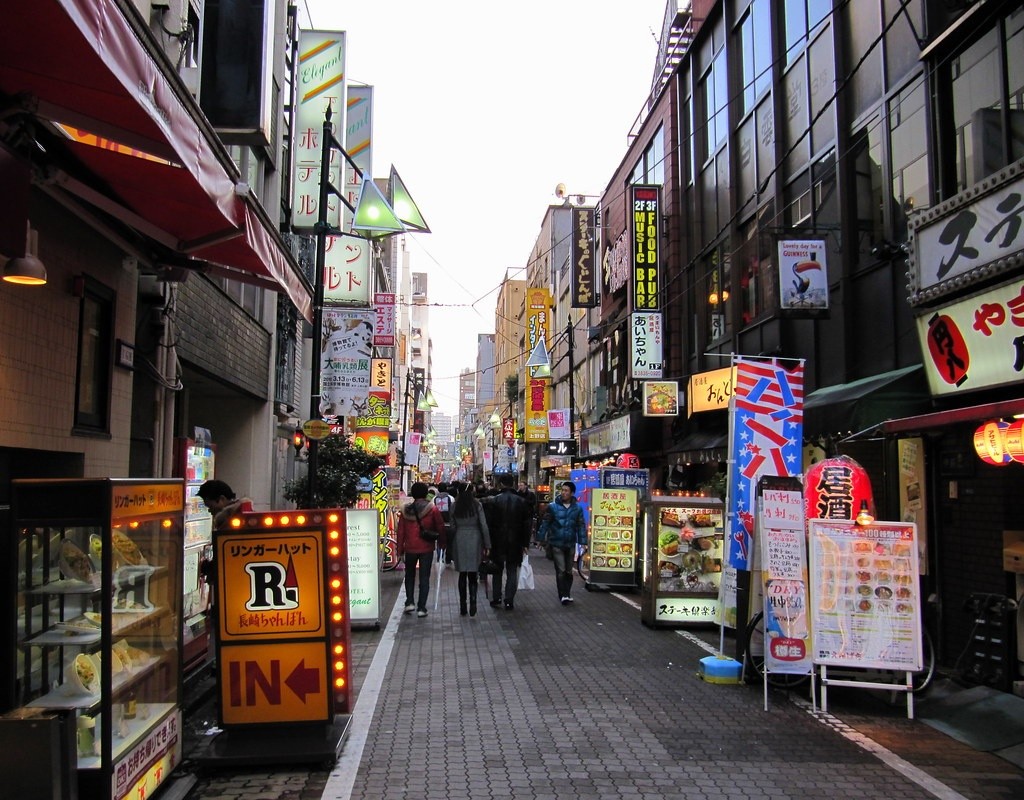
75;715;97;757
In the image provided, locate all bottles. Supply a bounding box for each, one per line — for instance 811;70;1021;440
124;690;137;719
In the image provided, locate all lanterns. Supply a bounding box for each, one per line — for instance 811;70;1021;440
616;453;640;469
1006;414;1024;464
801;456;874;539
973;417;1013;466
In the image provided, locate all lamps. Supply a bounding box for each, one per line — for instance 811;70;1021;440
292;419;308;464
2;217;49;286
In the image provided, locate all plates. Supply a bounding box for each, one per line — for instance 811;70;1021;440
814;542;914;616
592;516;633;568
656;530;722;593
53;610;114;633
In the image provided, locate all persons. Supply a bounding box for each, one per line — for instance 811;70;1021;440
196;479;254;582
538;481;587;603
396;474;535;618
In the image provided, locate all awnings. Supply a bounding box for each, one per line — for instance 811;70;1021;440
0;0;312;325
802;364;933;447
665;431;728;464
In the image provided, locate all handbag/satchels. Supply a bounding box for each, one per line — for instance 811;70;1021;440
517;553;534;590
420;526;439;540
478;553;497;574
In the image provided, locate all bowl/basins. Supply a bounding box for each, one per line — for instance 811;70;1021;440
63;644;133;696
59;528;148;585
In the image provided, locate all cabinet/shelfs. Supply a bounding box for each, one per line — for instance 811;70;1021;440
3;474;189;800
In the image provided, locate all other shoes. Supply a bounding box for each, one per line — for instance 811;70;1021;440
568;597;574;602
470;599;477;616
561;597;570;604
461;602;467;616
505;601;513;609
490;598;501;605
418;611;428;617
405;605;415;612
